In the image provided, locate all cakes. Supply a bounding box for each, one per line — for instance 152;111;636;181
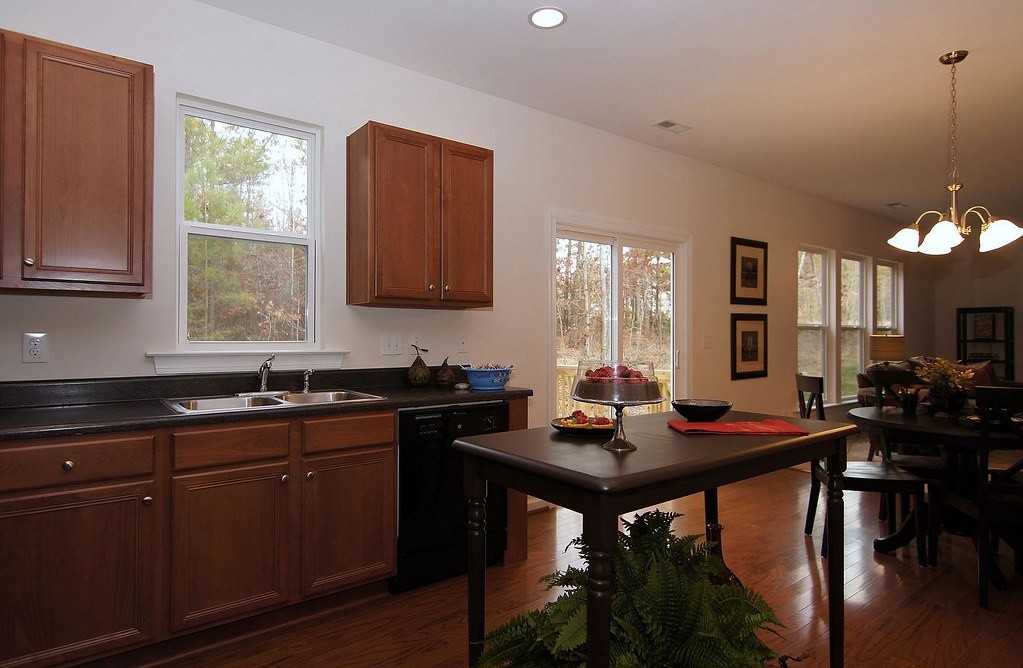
574;366;661;401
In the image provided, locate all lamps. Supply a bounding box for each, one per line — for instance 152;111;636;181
888;50;1023;254
870;334;909;371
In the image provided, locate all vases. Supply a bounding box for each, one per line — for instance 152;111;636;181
926;390;959;414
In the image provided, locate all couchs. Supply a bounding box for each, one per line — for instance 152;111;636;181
857;358;994;407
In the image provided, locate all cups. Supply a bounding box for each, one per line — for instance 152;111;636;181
903;394;918;417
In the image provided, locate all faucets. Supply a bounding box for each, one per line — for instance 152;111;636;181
303;368;314;393
258;354;277;393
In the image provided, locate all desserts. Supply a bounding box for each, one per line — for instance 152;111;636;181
560;410;613;429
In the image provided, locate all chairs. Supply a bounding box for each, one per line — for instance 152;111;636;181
793;371;1023;608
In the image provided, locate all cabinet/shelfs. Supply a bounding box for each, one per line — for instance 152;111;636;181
0;412;400;668
956;305;1015;381
346;120;495;308
0;28;153;301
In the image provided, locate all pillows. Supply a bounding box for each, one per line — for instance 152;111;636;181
863;360;993;387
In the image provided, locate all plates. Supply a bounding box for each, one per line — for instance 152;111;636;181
551;418;617;438
967;415;1023;425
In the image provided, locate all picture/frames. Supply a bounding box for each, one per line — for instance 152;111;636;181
730;237;767;305
729;314;768;379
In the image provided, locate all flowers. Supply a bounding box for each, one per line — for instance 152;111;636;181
916;358;977;392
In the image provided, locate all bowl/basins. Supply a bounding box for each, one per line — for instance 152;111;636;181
671;399;733;422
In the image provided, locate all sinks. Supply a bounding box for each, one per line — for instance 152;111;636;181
178;396;283;411
278;391;376;404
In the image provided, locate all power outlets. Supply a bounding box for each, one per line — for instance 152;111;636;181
22;332;48;363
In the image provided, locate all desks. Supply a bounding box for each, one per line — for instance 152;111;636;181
847;407;1022;609
455;410;855;668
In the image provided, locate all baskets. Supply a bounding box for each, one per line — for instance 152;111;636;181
462;367;512;392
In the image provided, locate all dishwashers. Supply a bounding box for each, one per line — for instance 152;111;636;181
385;399;509;597
